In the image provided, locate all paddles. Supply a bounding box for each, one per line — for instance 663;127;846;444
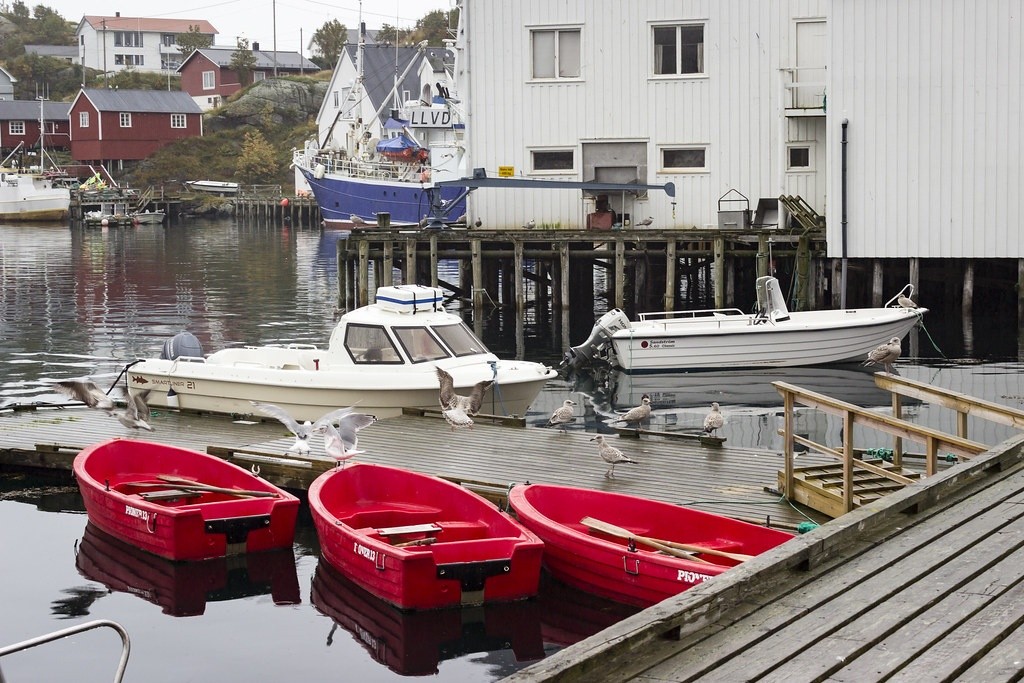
156;474;279;498
126;482;273;498
646;537;757;562
392;537;437;547
578;515;715;565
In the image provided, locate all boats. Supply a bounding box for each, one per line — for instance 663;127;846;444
132;212;167;224
310;551;547;679
286;0;469;227
505;478;802;607
0;98;71;222
72;437;300;561
554;282;929;382
71;521;304;617
606;367;929;416
185;180;238;193
527;577;636;649
101;281;558;425
378;139;429;162
305;461;547;612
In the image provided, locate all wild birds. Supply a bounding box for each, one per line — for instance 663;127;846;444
898;294;918;309
858;337;903;372
697;402;725;437
602;398;654;429
545;399;578;433
435;365;495;432
589;434;637;478
57;380;116;411
105;385;156;432
247;398;379;473
